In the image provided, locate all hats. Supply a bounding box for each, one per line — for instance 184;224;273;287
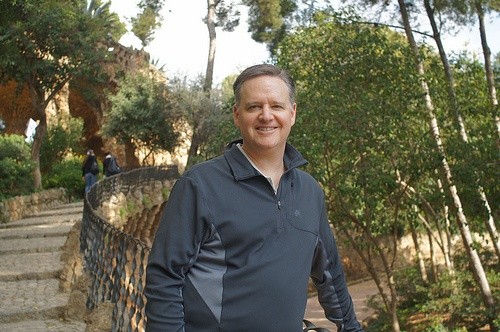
87;149;95;156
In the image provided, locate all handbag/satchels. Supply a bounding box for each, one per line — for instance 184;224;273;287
107;158;121;174
91;162;100;176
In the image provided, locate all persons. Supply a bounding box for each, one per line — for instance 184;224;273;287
81;149;100;194
142;63;364;332
102;150;123;178
